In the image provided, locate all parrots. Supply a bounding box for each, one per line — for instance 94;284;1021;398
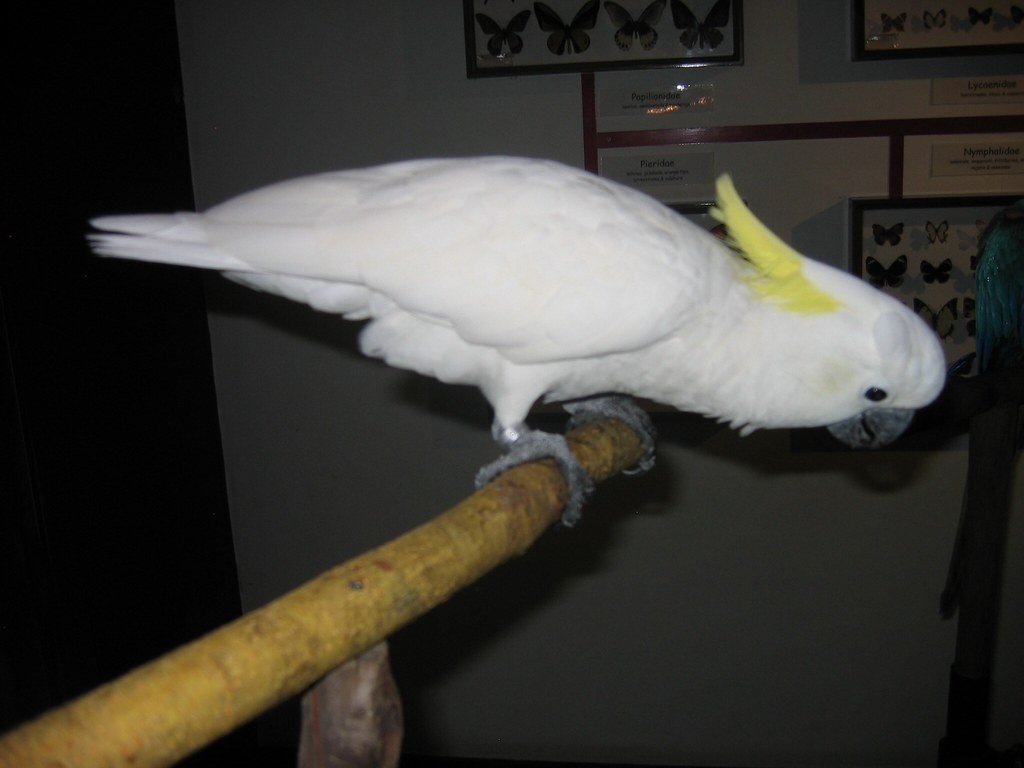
88;153;949;525
